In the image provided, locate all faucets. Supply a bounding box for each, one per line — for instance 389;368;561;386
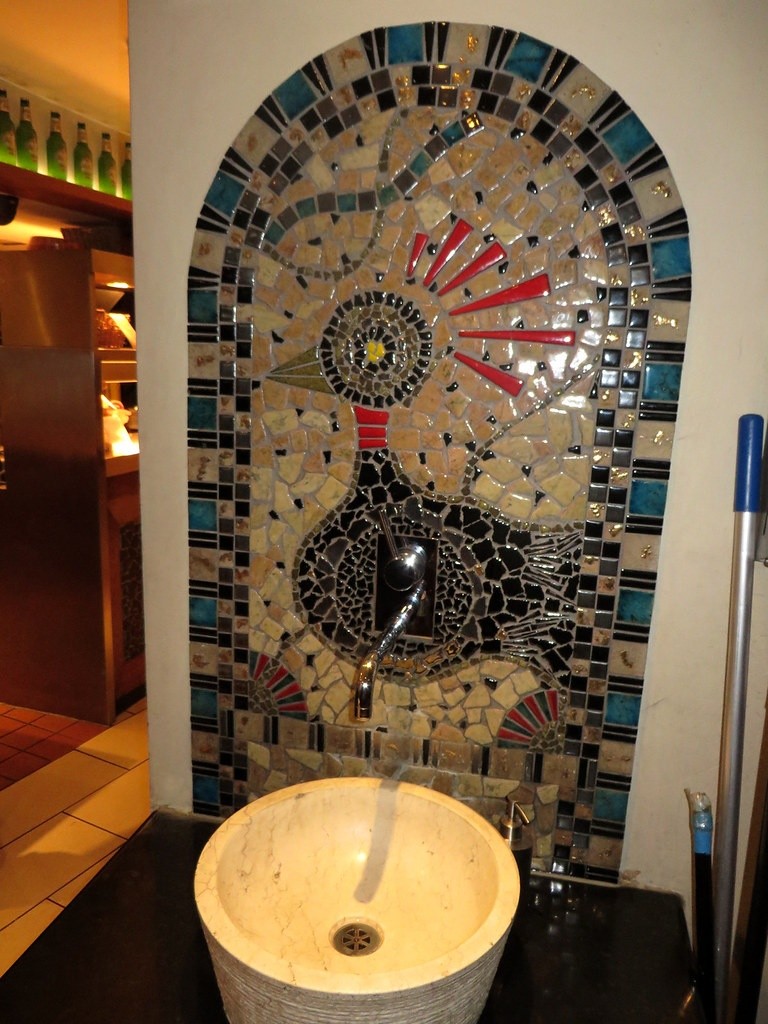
353;587;423;722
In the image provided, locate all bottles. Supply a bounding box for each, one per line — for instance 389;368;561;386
0;85;131;207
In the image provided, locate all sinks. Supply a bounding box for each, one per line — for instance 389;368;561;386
192;776;522;1024
